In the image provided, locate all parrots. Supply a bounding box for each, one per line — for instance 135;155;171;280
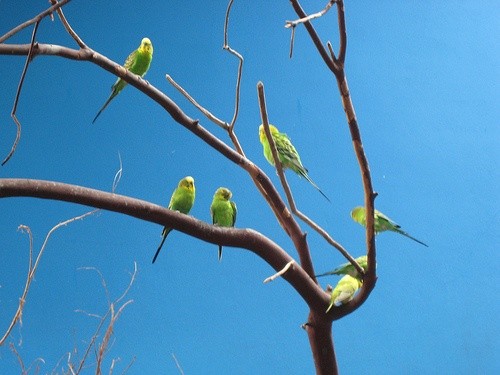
92;37;154;123
152;175;196;264
351;206;429;248
258;123;332;201
209;187;238;261
312;255;368;314
